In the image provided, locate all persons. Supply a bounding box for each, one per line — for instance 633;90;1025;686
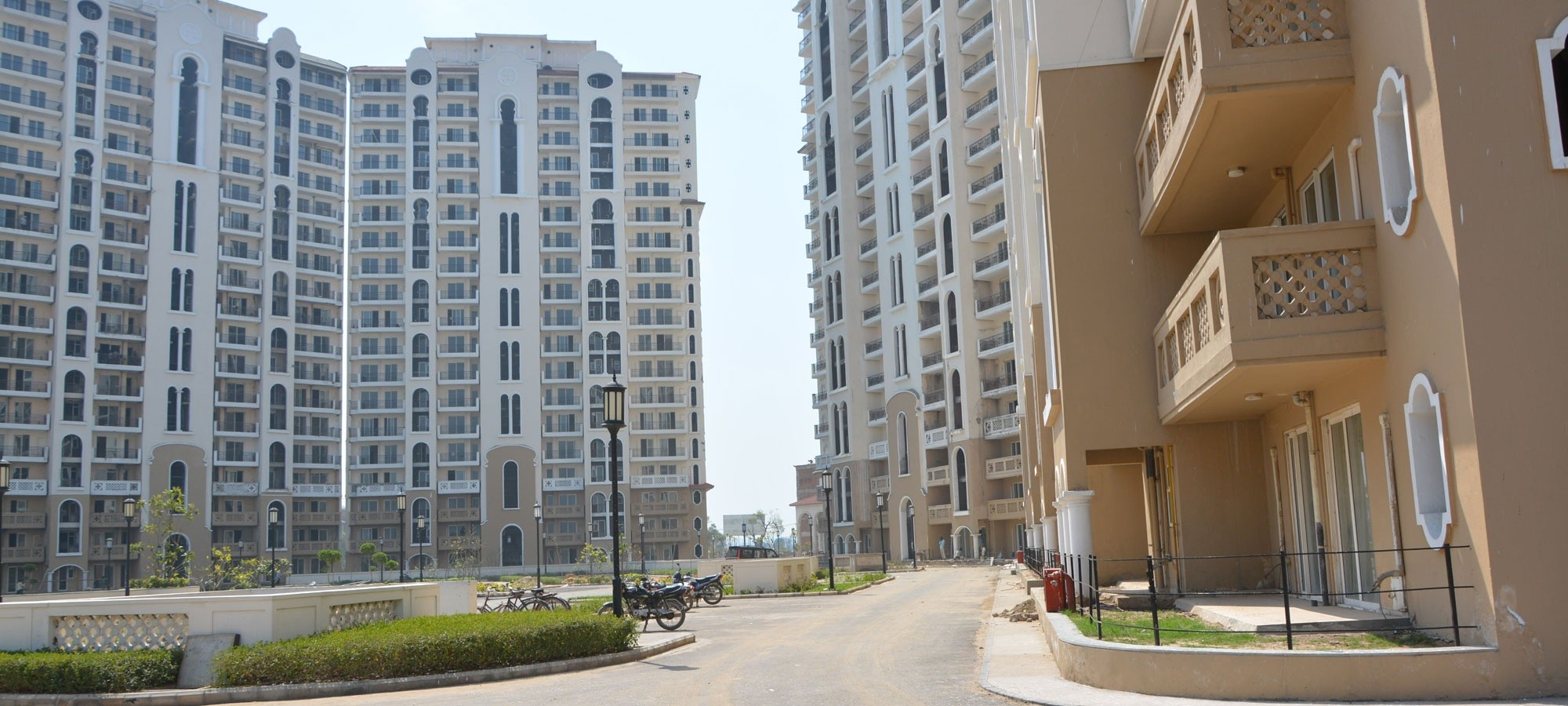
16;580;26;595
938;537;948;560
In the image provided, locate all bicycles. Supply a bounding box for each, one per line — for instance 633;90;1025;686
502;583;571;613
477;587;553;615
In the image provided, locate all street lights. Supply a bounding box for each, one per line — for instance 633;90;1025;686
586;520;593;576
821;465;835;589
639;511;646;573
238;539;243;564
875;488;887;574
379;537;384;583
106;538;113;591
269;507;279;588
532;500;542;589
417;514;425;582
397;487;407;582
698;517;813;561
542;530;548;574
602;370;627;617
909;503;916;569
123;496;137;596
0;458;11;604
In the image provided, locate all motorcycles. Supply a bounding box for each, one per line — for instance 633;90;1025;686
672;563;728;606
637;576;694;611
598;577;686;633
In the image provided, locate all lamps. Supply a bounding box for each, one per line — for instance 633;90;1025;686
1245;393;1263;401
1226;166;1245;178
